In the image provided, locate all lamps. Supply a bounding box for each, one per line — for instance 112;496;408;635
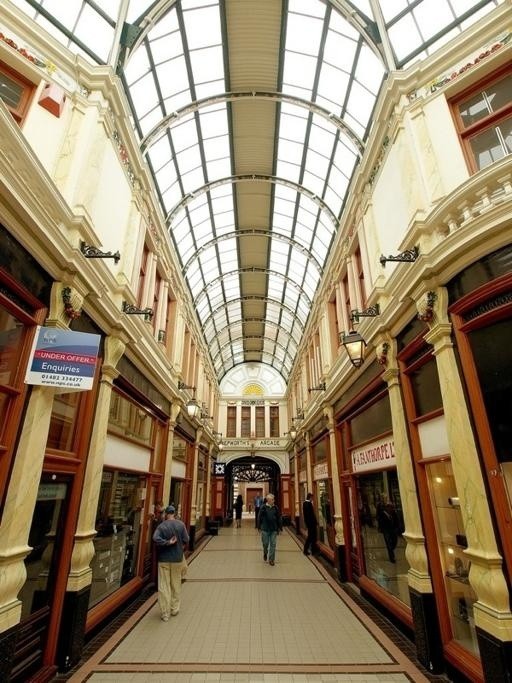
287;413;305;444
341;302;380;368
179;380;201;421
211;430;224;454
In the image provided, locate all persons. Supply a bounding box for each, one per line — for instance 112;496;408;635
150;504;191;623
232;493;243;529
301;491;321;555
255;492;283;567
149;500;189;582
253;490;264;529
374;501;403;564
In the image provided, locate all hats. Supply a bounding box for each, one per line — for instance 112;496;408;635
164;506;176;513
